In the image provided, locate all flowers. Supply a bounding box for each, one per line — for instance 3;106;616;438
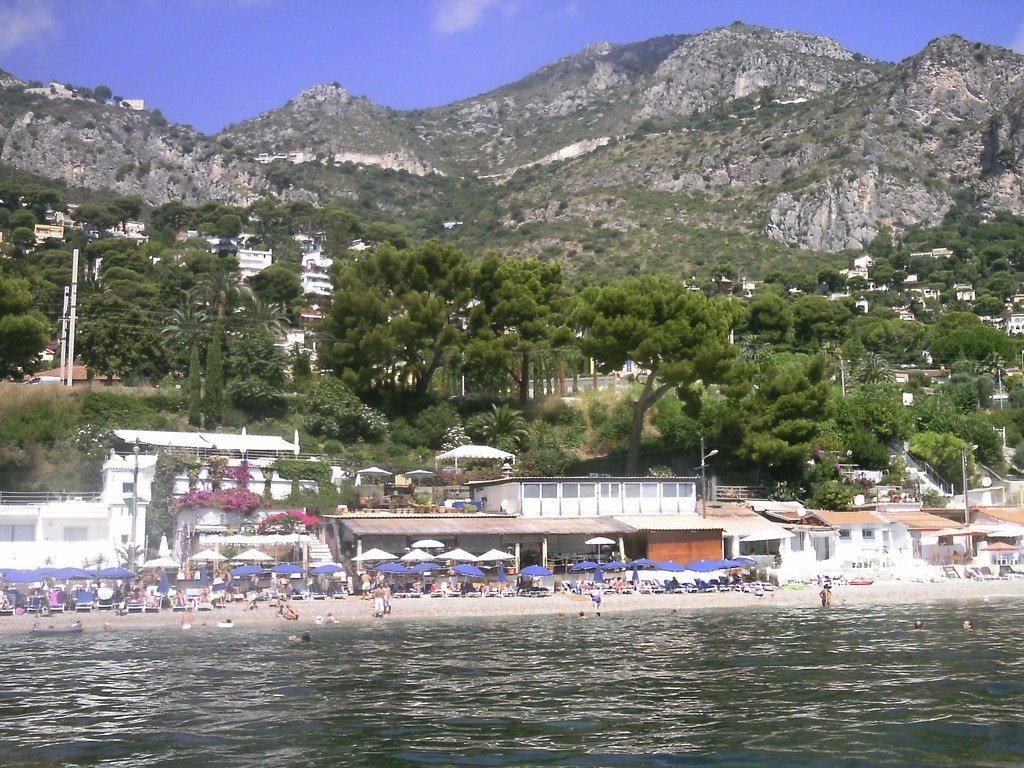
146;454;323;535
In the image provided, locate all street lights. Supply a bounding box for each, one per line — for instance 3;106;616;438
694;437;719;519
960;443;980;525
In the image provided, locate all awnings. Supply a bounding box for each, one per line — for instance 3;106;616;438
740;525;795;568
357;467;393;485
434;444;515;475
112;428;295;459
406;469;434;486
0;536;757;613
933;526;1023;581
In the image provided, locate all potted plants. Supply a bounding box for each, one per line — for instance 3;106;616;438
338;487;481;515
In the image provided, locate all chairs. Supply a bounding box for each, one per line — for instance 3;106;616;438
0;561;1023;615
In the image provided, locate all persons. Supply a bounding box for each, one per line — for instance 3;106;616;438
963;620;974;631
4;569;657;630
820;584;828;607
826;586;832;606
670;577;678;590
910;620;930;632
292;633;310;642
671;609;677;615
